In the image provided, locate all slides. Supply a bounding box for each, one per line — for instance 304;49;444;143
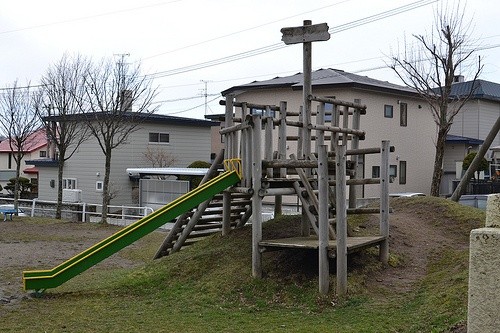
23;171;240;290
